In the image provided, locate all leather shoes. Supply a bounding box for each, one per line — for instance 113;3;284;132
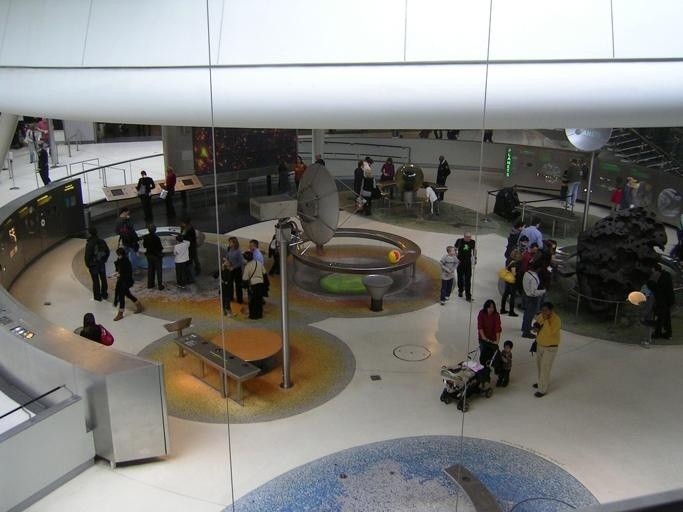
533;384;539;388
522;331;536;338
500;309;508;313
496;379;503;387
502;379;509;386
535;391;546;397
509;311;518;316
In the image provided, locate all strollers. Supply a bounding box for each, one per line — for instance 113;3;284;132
437;342;501;413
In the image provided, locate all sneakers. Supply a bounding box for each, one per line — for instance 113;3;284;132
445;296;449;300
440;301;445;305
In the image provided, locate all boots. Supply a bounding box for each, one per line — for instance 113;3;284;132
133;302;144;314
147;281;156;288
158;281;164;290
113;311;124;321
137;179;146;196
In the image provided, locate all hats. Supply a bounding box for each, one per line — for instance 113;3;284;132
147;224;156;232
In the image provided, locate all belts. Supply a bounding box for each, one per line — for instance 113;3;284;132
544;345;558;348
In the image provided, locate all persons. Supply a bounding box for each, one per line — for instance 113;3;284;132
439;232;477;305
423;155;451;214
559;159;582;207
650;264;676;340
85;207;201;322
135;168;176;222
476;299;502;371
611;175;640;212
416;130;494;143
354;157;394;216
26;118;51;186
73;312;114;346
501;218;557;338
646;279;673;339
279;153;325;191
220;234;280;320
495;340;513;387
531;301;561;397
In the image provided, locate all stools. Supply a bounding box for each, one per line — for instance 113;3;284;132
361;272;393;311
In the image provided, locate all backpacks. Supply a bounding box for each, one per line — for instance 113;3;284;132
92;240;108;264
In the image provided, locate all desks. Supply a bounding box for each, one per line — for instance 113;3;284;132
528;204;579;239
376;179;449;222
212;326;284;370
289;226;422;297
126;224;205;270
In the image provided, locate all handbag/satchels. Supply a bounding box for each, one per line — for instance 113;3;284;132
529;341;537;352
236;280;249;290
497;261;517;284
98;324;114;346
160;189;168;199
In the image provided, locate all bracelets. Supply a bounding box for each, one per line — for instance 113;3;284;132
485;337;491;343
